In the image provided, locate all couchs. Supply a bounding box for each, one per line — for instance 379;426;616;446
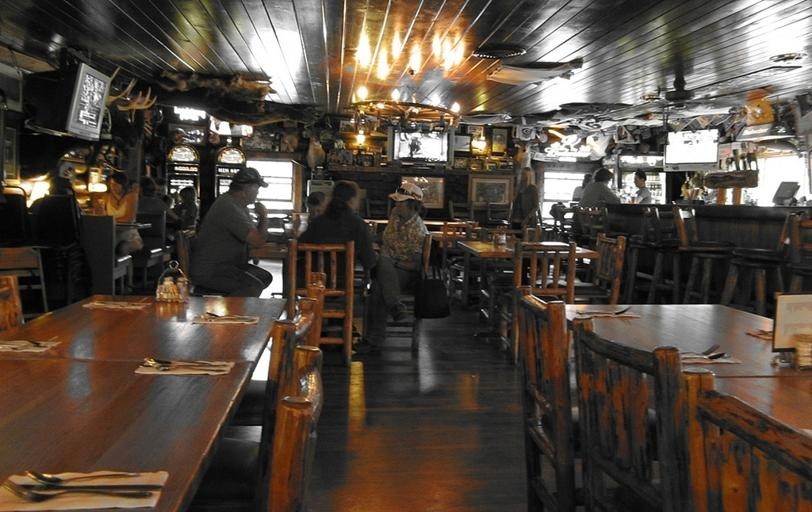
132;210;167;291
80;215;132;294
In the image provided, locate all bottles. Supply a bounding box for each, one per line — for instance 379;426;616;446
161;276;190;305
495;230;507;246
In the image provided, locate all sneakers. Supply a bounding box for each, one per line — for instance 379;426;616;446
352;339;374;355
390;302;407;321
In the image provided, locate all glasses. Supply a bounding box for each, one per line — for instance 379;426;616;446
395;187;417;201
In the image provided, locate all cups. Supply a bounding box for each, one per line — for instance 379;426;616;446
284;223;293;237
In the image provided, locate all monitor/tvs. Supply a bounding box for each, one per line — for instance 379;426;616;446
25;59;112;143
387;122;455;169
663;128;720;173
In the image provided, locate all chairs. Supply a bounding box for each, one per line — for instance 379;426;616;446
364;234;432;352
366;198;391;218
0;246;49;319
487;203;514;220
448;201;473;220
556;203;812;313
0;275;24;332
443;221;572;361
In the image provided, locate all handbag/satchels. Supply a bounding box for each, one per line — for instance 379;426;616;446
521;225;542;241
414;277;450;318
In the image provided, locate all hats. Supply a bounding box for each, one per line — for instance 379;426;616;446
388;183;424;202
636;168;646;180
233;168;268;188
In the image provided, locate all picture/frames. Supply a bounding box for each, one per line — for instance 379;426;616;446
467;173;514;209
401;176;445;208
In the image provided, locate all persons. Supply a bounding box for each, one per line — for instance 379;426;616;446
508;168;539;285
353;181;429;356
304;190;326;222
572;174;592;201
188;168;273;297
29;171;197;313
574;166;620;234
627;170;652;204
287;179;378;356
